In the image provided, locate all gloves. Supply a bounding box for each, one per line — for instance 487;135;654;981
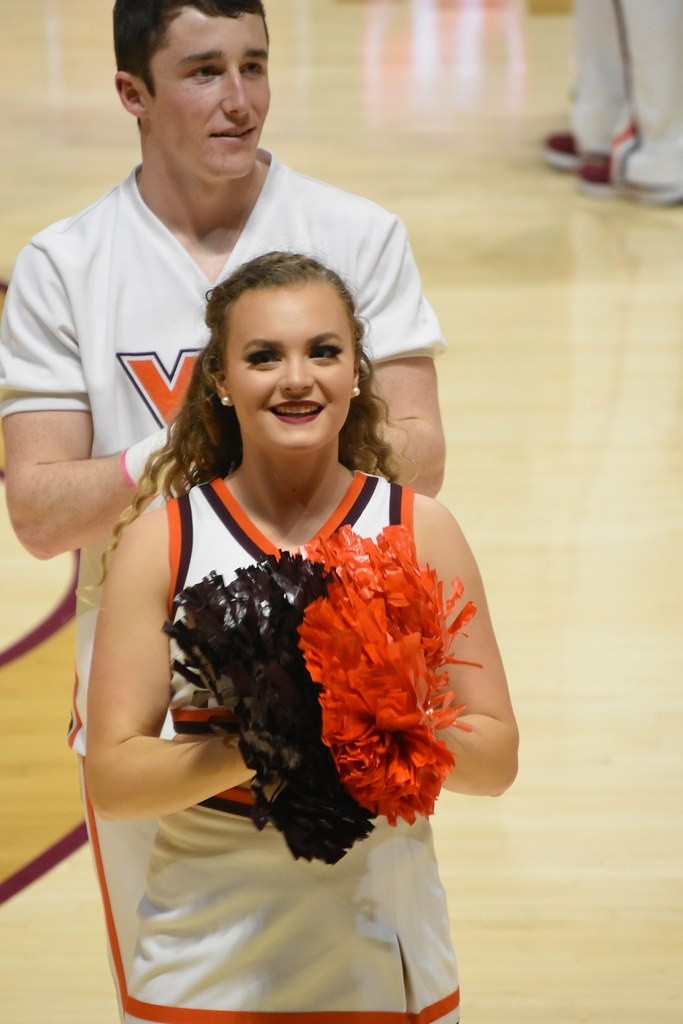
120;423;174;490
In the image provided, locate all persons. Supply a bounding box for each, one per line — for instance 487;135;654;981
82;247;520;1024
1;0;442;1024
536;0;683;204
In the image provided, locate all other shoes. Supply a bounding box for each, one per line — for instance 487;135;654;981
540;132;581;169
578;158;614;197
